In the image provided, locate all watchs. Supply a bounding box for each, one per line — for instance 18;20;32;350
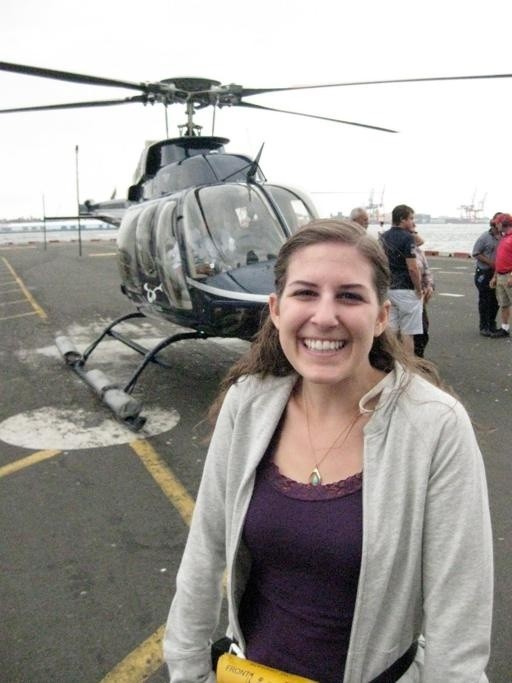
209;263;215;270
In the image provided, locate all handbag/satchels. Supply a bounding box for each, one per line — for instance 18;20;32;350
216;651;318;683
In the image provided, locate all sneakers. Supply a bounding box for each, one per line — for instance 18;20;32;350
480;325;508;337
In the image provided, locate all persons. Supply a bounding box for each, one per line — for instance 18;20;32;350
473;212;499;337
165;216;496;683
487;213;512;338
349;205;434;360
167;204;230;281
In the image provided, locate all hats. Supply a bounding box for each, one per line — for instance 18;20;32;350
491;212;512;226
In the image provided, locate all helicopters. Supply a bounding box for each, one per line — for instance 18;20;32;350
0;59;511;433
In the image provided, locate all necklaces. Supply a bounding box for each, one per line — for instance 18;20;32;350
303;385;368;485
